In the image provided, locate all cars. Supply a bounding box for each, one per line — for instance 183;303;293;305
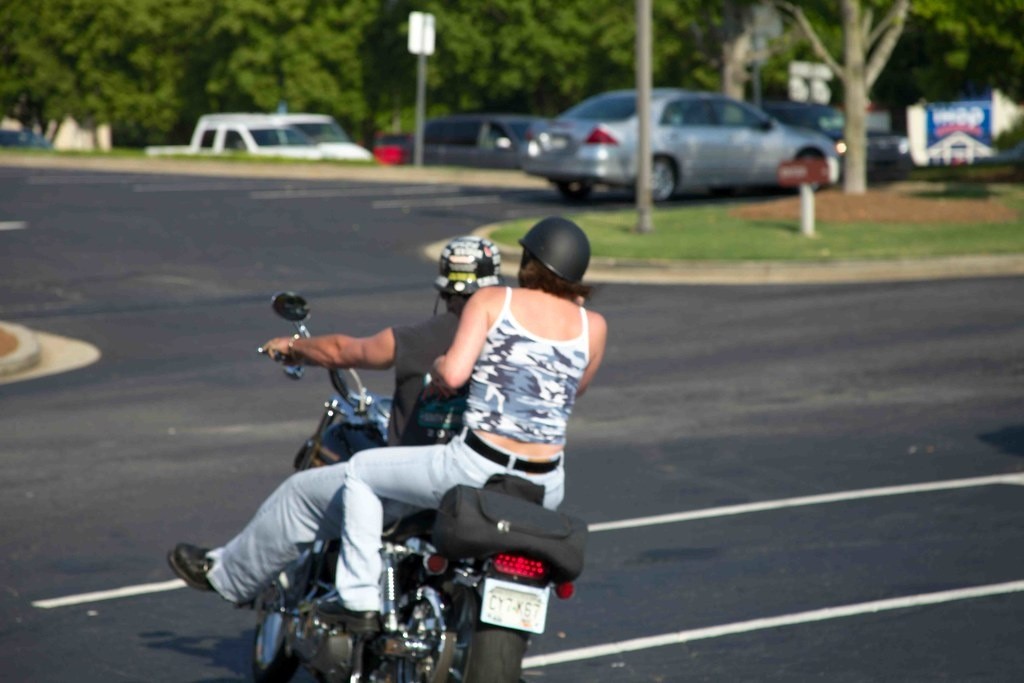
523;88;839;205
370;117;552;172
756;99;912;187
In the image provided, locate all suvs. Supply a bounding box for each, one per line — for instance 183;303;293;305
270;113;382;160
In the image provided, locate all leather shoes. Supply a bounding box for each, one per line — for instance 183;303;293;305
314;595;380;634
168;542;217;593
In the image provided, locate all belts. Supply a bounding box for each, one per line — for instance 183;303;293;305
464;428;560;473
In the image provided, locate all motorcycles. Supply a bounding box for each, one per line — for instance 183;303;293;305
228;288;575;683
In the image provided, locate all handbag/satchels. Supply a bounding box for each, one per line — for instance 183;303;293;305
433;473;588;585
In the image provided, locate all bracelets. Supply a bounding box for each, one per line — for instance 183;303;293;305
288;334;305;364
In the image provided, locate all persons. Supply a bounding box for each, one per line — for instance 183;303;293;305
165;235;509;605
314;213;609;639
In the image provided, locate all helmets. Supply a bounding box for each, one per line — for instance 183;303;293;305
434;236;501;295
519;217;590;283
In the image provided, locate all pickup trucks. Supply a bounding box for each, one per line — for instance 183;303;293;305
143;112;332;160
